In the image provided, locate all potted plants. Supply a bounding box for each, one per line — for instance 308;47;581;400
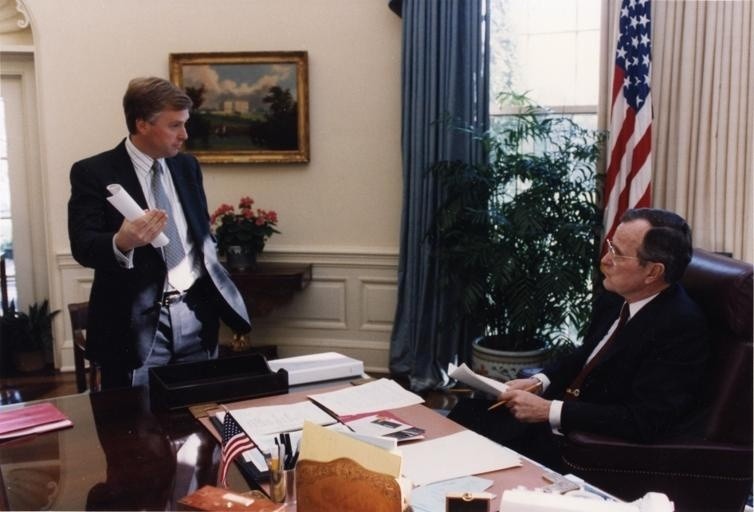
419;89;610;405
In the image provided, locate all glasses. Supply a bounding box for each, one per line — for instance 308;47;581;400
606;238;654;265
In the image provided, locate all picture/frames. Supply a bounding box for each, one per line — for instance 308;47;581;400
167;51;312;164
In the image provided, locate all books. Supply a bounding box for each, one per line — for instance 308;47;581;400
266;350;366;386
0;402;74;442
339;410;426;443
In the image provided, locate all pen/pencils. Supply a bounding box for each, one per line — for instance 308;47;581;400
271;433;300;497
487;382;543;411
542;475;556;485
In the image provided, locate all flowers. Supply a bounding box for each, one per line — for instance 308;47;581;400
208;196;282;259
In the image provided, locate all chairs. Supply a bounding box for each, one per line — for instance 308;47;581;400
450;248;753;512
68;301;100;392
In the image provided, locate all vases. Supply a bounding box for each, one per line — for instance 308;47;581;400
226;246;257;274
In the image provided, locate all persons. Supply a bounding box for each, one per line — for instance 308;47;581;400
66;76;254;393
444;205;714;494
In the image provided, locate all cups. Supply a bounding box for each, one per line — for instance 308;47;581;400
267;465;297;506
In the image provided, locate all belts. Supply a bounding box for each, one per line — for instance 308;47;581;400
165;293;197;306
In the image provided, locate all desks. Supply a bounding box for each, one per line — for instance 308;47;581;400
186;367;654;512
218;262;313;323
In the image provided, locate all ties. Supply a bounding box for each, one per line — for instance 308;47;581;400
151;157;190;294
562;303;629;401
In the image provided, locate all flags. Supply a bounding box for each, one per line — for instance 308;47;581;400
218;413;258;490
602;0;657;255
